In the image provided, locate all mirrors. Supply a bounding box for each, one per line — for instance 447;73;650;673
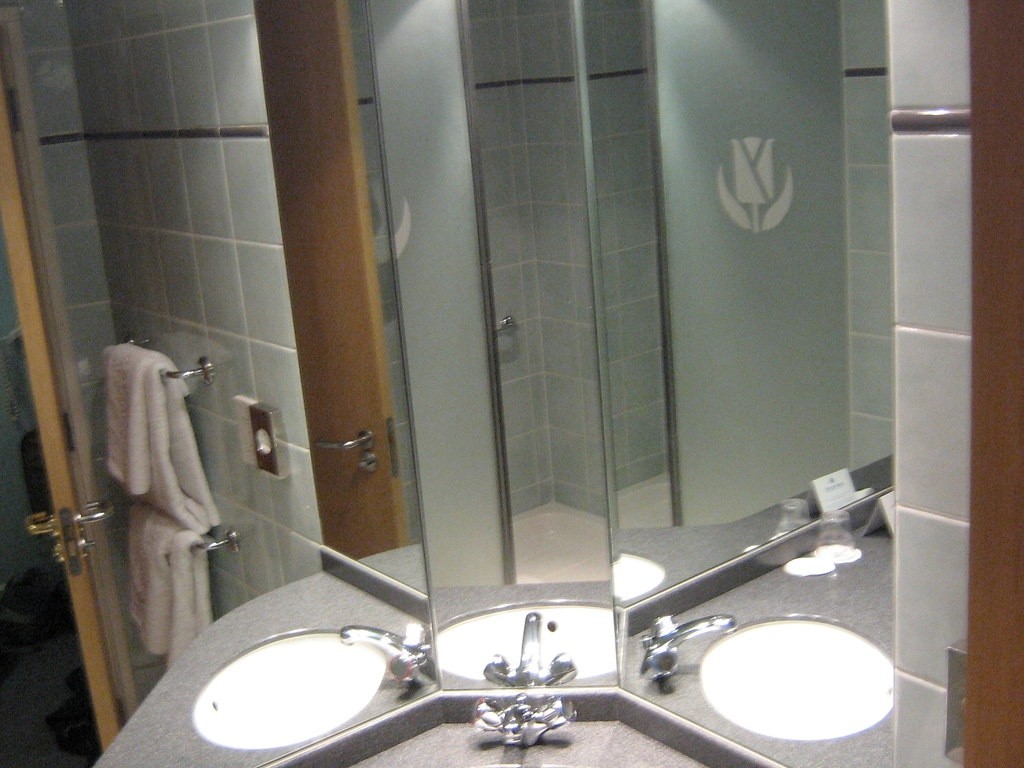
366;0;622;725
572;0;898;768
0;0;445;768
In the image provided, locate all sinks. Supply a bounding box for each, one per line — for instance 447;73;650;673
188;626;389;753
435;598;617;681
694;612;895;743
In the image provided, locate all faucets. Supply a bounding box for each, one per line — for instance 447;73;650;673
637;613;739;680
337;623;434;673
499;693;533;748
513;610;544;687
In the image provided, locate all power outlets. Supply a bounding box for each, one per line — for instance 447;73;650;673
233;394;259;467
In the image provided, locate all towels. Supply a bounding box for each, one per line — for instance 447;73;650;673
124;501;215;672
100;341;223;536
